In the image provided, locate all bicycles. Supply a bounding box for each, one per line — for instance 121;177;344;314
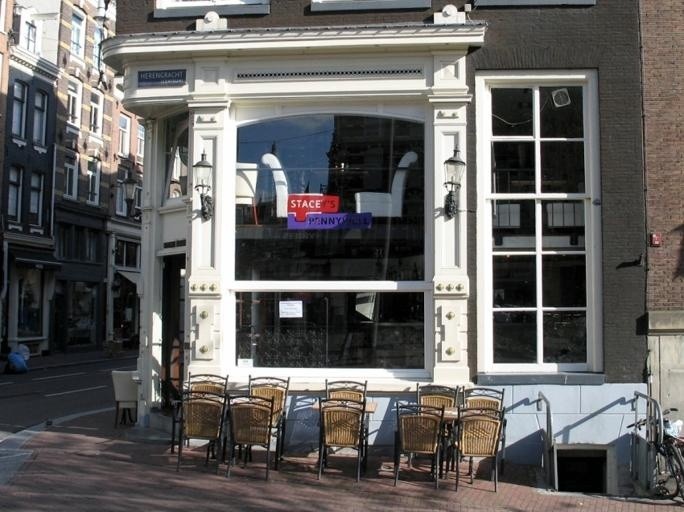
626;405;684;503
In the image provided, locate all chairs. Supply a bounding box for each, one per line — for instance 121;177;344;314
353;149;418;219
172;372;291;481
408;382;507;479
316;379;377;482
445;402;506;493
394;400;446;489
235;161;263;224
261;152;323;219
110;370;139;427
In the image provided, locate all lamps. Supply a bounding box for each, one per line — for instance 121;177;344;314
442;145;466;218
190;145;214;220
117;164;142;220
